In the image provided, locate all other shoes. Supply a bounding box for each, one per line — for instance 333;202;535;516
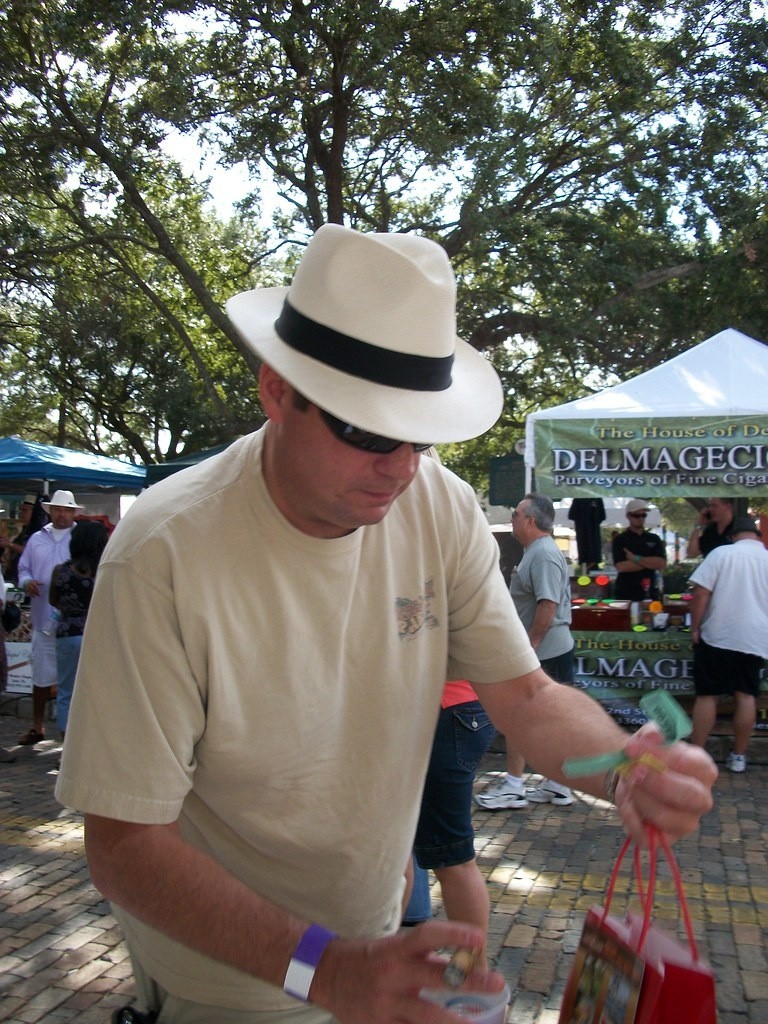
18;728;44;745
0;748;17;762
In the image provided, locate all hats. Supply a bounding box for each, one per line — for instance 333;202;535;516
19;495;37;510
728;517;763;537
41;489;85;514
625;499;651;515
224;222;504;443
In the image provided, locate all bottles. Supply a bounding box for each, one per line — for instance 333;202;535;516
631;570;663;633
41;607;61;636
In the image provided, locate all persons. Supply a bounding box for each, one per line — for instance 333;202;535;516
692;516;768;772
688;499;738;560
414;683;496;939
0;495;37;692
48;521;110;739
604;532;619;566
509;494;577;687
17;490;86;747
610;500;667;604
55;222;718;1024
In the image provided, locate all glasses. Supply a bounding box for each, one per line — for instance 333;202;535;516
511;510;532;520
320;409;433;454
627;512;647;518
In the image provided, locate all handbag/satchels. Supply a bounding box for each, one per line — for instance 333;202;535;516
558;821;719;1024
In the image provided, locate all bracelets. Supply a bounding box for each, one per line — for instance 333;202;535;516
283;920;341;1001
8;542;14;547
634;555;640;562
23;580;30;588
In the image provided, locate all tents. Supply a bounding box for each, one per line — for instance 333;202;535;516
1;435;148;497
148;438;236;485
523;327;768;526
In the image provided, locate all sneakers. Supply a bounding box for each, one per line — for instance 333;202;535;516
526;779;574;805
473;778;529;809
724;751;747;772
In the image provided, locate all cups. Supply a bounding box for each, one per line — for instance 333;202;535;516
653;613;668;631
419;976;511;1024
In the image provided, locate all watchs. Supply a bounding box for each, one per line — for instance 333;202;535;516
603;767;621;804
